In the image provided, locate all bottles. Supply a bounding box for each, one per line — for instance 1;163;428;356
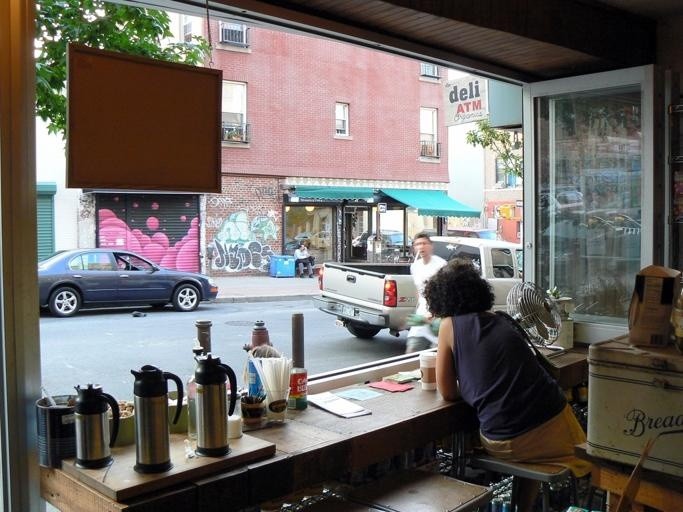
225;390;242;440
571;382;588;433
488;475;513;511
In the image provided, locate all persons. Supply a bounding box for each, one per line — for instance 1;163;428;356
403;233;448;352
426;257;585;510
293;244;314;278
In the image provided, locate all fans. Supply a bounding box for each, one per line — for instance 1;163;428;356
503;279;566;360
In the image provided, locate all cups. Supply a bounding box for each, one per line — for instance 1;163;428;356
34;395;76;468
166;390;189;435
544;317;574;350
107;401;135;447
265;387;291;423
419;352;437;392
240;396;264;424
247;360;264;396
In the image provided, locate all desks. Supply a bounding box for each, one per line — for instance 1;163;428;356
43;336;591;512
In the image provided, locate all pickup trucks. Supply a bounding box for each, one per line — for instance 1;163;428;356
310;236;526;342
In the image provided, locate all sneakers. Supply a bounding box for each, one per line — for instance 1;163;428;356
309;274;314;278
300;274;305;278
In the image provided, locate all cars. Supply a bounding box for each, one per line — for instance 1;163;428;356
353;226;505;256
537;183;641;285
284;226;331;254
36;246;217;318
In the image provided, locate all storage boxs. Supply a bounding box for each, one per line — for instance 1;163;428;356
585;330;682;480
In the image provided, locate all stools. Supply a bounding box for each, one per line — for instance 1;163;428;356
293;456;572;512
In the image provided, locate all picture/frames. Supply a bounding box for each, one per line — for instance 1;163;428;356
62;42;223;194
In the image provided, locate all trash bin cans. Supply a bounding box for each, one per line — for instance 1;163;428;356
269;255;296;278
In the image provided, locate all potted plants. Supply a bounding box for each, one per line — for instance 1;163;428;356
545;286;572;350
